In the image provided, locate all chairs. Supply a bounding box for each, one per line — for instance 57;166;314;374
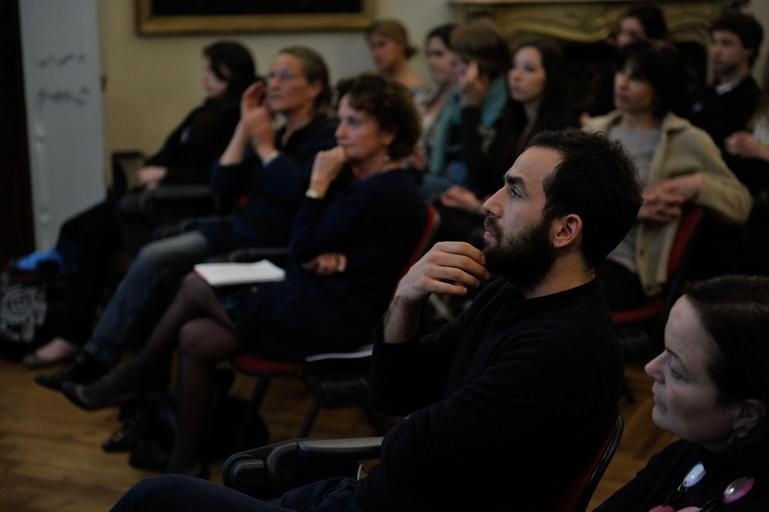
56;196;708;511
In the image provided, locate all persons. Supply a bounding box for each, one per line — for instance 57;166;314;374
3;6;769;512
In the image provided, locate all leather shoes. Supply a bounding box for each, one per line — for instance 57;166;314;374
22;341;167;454
